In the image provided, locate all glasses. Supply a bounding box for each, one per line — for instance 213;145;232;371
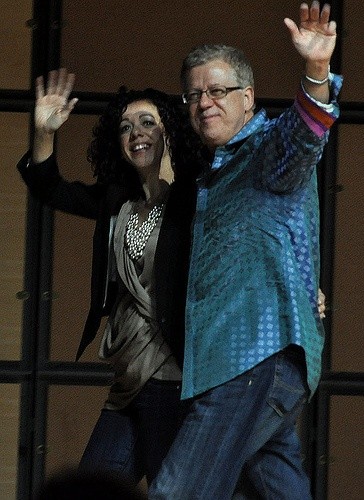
180;83;247;105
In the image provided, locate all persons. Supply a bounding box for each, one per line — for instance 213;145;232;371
17;68;327;489
143;0;345;499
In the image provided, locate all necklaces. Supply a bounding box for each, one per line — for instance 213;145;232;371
126;179;176;260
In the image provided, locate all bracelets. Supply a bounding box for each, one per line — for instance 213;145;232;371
303;68;330;84
